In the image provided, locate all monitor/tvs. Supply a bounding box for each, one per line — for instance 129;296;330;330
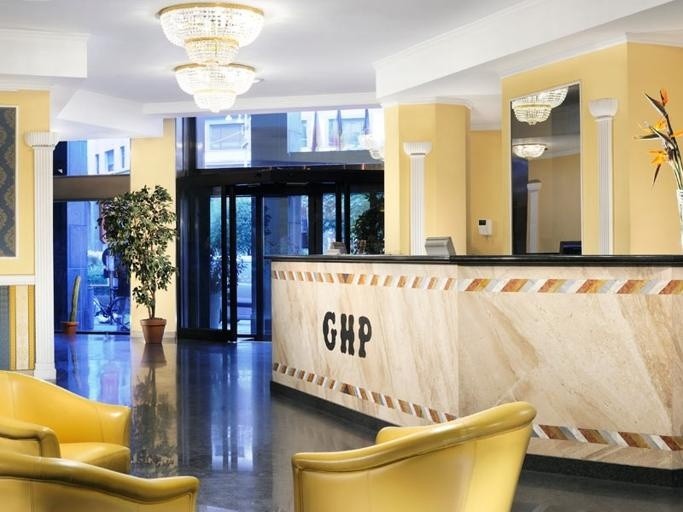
560;241;581;255
425;236;457;255
330;242;348;254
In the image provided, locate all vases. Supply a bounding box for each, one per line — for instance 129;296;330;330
61;275;81;333
676;189;683;248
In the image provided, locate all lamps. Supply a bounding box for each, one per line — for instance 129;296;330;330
512;144;547;162
154;2;265;69
512;88;569;126
171;63;258;115
633;90;683;190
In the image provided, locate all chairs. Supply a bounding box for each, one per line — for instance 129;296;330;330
292;401;537;512
0;453;199;512
0;369;133;475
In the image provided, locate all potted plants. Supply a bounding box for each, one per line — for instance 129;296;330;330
61;333;81;387
97;186;182;344
127;344;177;479
